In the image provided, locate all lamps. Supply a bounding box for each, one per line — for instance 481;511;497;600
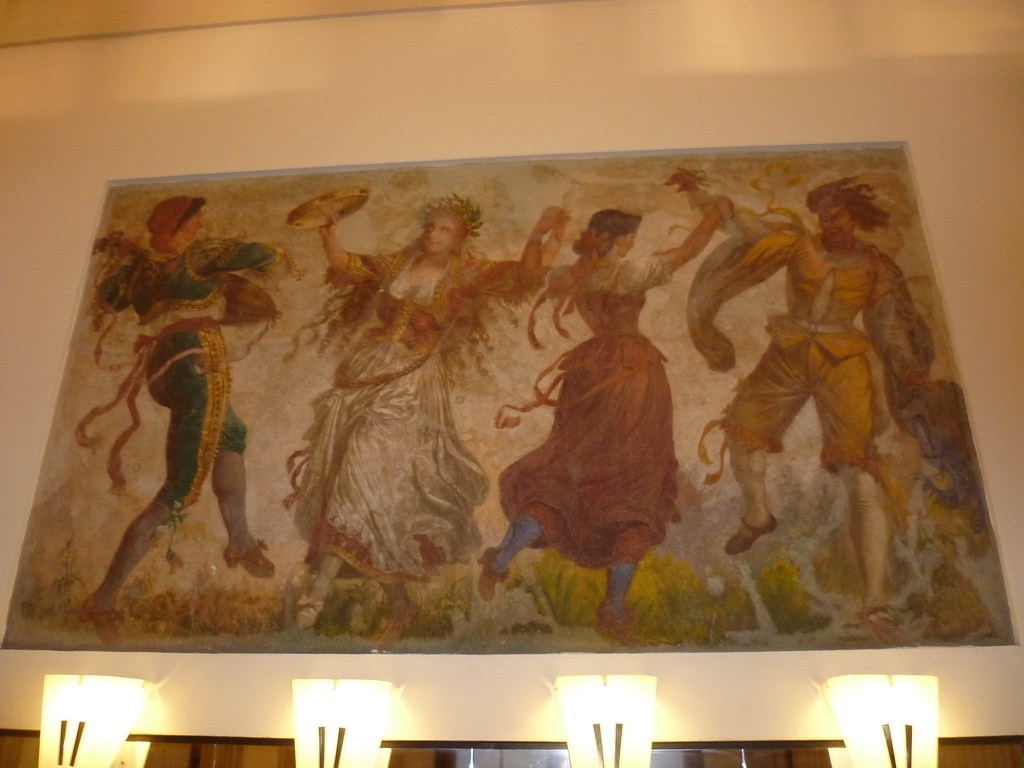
555;673;656;768
35;676;153;768
293;678;394;768
826;673;938;768
